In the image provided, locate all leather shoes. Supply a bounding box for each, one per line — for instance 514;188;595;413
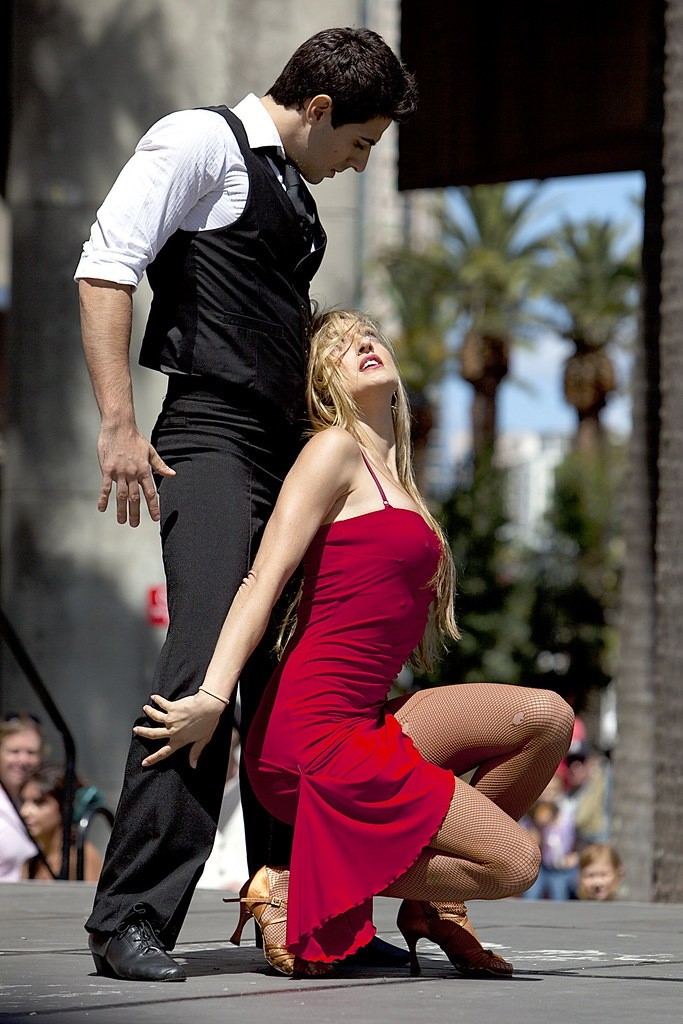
89;915;188;980
254;916;411;969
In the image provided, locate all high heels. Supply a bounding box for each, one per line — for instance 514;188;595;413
398;897;515;979
230;865;334;976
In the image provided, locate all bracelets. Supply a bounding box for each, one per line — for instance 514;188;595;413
198;686;231;706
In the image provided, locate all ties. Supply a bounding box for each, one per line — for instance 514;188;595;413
283;160;312;254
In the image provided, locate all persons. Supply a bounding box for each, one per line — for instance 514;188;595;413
0;715;56;883
71;28;428;983
16;766;103;884
129;309;578;982
517;717;626;902
